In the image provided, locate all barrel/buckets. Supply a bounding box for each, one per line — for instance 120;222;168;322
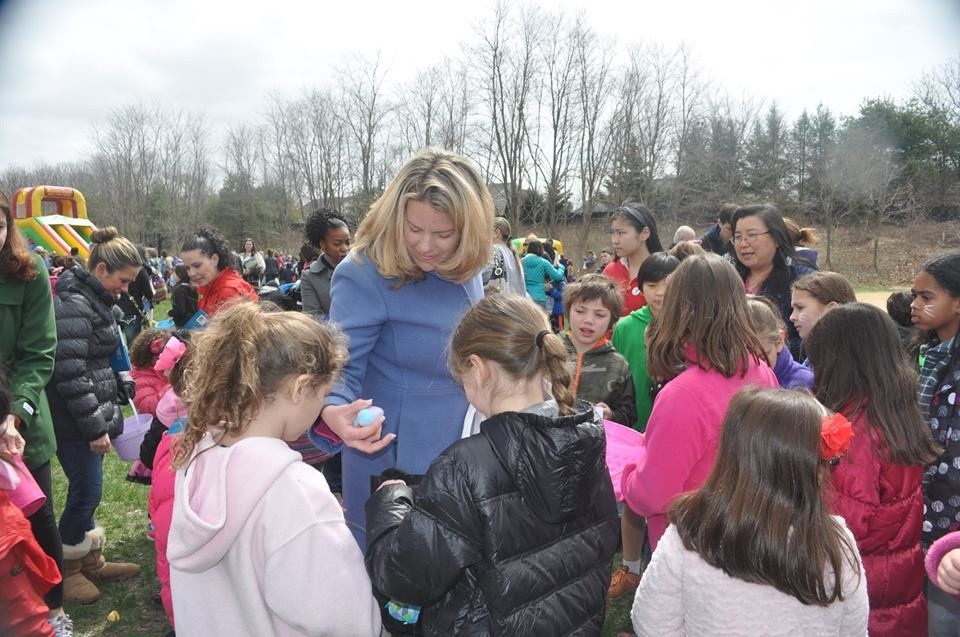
110;398;153;462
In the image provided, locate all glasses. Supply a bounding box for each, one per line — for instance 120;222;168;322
730;230;770;246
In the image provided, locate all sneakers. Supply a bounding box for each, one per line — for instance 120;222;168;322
606;564;641;600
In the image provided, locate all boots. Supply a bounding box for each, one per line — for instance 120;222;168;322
80;527;140;581
61;531;100;603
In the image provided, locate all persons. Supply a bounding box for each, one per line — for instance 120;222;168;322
740;293;818;429
0;191;696;637
608;252;685;604
789;270;863;403
912;252;960;468
923;439;960;637
309;147;534;590
620;252;780;562
541;272;643;430
884;288;925;371
703;202;835;365
801;301;929;637
365;291;618;636
630;387;871;637
601;200;662;325
166;295;393;637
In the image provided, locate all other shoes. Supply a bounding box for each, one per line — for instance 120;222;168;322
47;614;74;637
151;592;163;608
146;523;155;541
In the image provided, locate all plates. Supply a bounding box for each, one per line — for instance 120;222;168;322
156;320;175;327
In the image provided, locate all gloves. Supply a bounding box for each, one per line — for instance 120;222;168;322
116;380;135;405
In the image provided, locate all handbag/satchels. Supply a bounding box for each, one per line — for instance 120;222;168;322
148;269;168;302
483;246;508;296
141;312;152;330
